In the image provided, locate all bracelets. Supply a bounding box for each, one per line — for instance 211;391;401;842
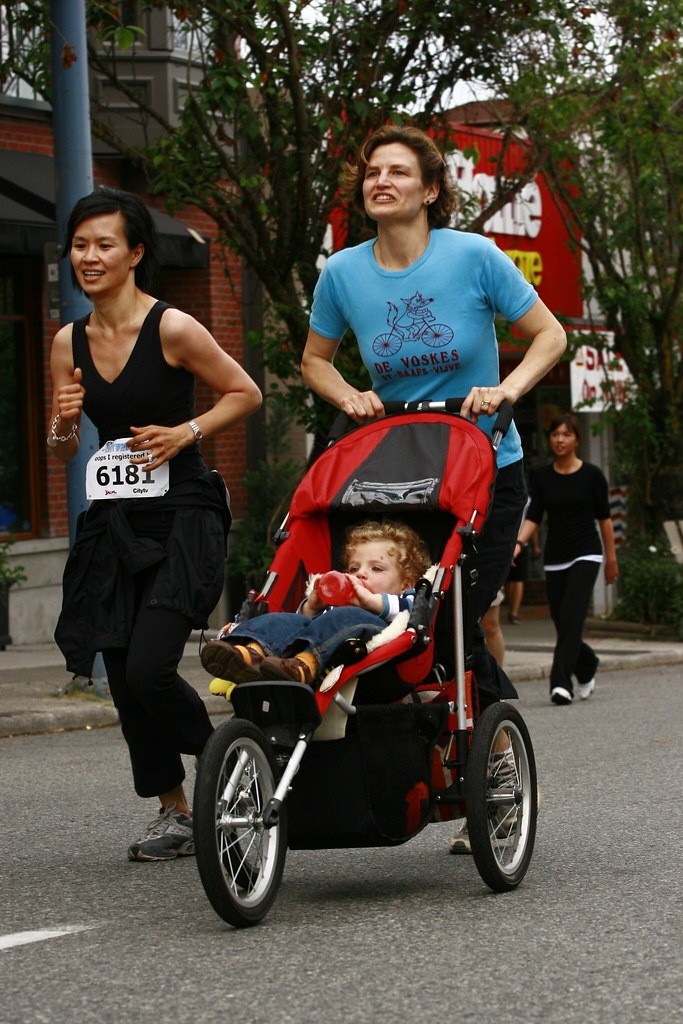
516;540;523;547
51;415;78;441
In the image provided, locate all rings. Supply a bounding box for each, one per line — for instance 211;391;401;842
148;454;153;462
481;401;490;406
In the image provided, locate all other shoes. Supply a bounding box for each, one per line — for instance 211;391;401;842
508;613;521;626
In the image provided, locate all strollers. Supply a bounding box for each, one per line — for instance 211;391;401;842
191;395;538;928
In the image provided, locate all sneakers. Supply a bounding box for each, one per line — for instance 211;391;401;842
491;749;518;839
576;677;595;700
551;687;572;705
127;801;195;861
449;821;473;854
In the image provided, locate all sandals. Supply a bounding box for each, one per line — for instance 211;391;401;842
260;655;313;688
201;641;265;684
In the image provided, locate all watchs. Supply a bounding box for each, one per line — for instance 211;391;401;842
188;420;205;441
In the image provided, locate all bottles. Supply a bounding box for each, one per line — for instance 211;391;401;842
316;571;371;607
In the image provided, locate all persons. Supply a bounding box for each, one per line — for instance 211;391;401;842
49;189;262;858
301;126;568;852
199;523;431;684
505;531;541;626
512;415;619;705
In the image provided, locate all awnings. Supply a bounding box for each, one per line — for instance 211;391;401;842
1;150;210;281
